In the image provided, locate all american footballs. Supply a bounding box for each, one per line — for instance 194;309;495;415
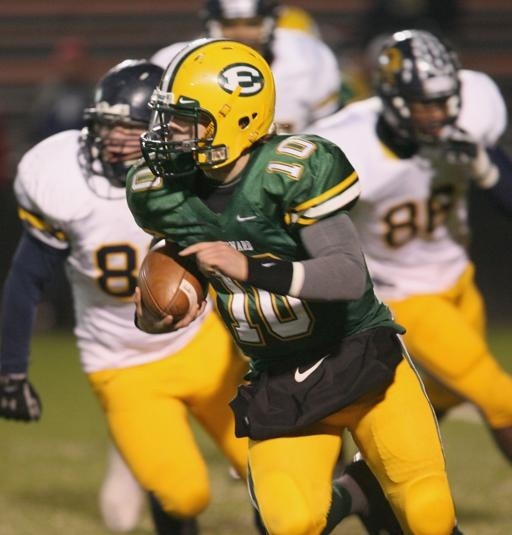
136;235;208;323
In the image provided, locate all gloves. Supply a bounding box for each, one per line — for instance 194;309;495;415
1;372;42;424
417;125;500;193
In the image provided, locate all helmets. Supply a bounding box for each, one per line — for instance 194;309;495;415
82;58;166;179
366;28;461;146
136;37;275;182
199;0;278;47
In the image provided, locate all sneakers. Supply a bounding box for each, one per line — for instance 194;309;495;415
343;451;403;534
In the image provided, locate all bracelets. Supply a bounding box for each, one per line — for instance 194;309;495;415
248;251;295;297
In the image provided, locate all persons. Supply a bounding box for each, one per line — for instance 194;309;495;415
95;41;244;533
0;58;251;534
207;0;342;138
300;28;510;466
124;37;465;534
